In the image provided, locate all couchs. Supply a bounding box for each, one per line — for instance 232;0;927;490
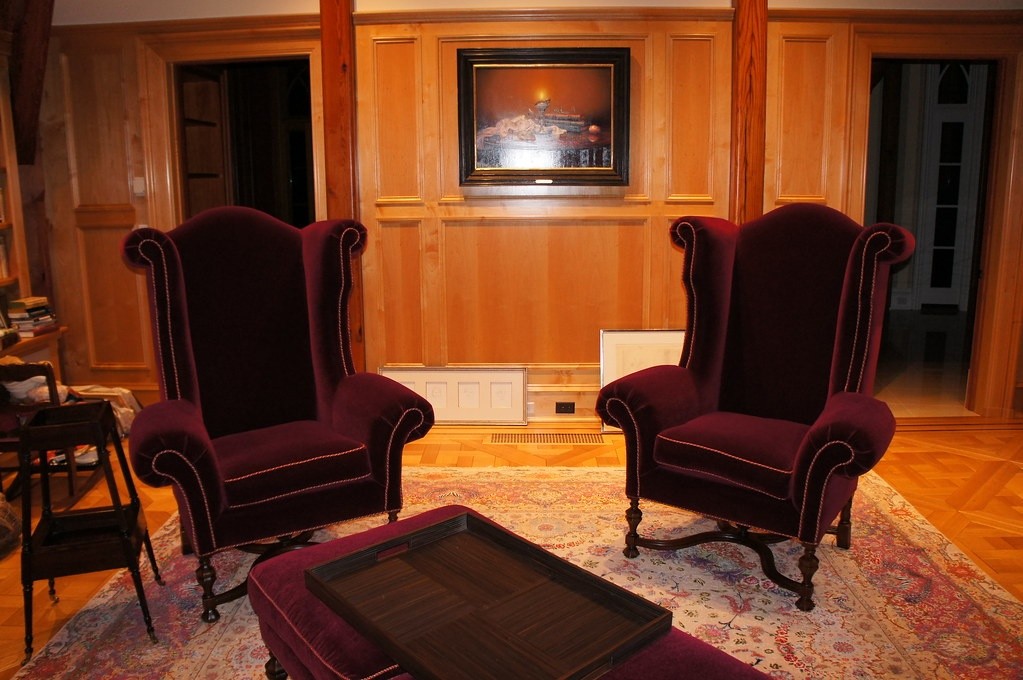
597;201;919;616
119;205;435;624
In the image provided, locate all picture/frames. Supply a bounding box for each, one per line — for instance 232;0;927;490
600;328;686;433
377;364;528;426
455;46;635;190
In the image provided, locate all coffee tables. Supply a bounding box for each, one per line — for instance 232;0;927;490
246;503;791;680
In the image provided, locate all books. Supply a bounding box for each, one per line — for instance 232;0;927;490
7;297;59;337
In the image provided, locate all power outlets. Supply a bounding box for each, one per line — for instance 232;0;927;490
556;401;576;414
527;402;535;414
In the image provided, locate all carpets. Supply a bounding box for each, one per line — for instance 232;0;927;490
10;462;1023;680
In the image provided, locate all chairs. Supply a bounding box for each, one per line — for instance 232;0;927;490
0;360;104;499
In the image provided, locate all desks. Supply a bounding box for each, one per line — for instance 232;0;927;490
0;324;70;386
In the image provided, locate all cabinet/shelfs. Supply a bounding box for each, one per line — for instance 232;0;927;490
0;54;33;300
175;60;226;219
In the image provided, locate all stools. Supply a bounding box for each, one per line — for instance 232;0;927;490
21;400;167;667
